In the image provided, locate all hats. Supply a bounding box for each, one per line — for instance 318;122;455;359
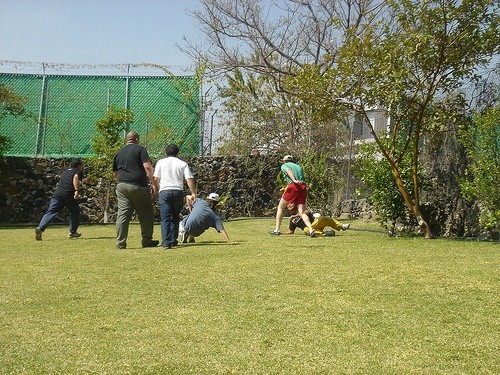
207;192;220;202
282;155;294;162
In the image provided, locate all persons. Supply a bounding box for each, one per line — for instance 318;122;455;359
267;154;316;237
112;131;159;249
281;203;351;236
34;158;84;242
178;193;234;245
149;144;196;249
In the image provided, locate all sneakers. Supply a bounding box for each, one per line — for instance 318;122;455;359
325;230;335;235
35;227;43;241
141;238;160;247
269;230;280;235
188;239;195;243
171;240;178;246
116;244;127;249
68;231;82;239
342;223;350;231
182;239;187;243
163;244;172;249
310;230;316;236
